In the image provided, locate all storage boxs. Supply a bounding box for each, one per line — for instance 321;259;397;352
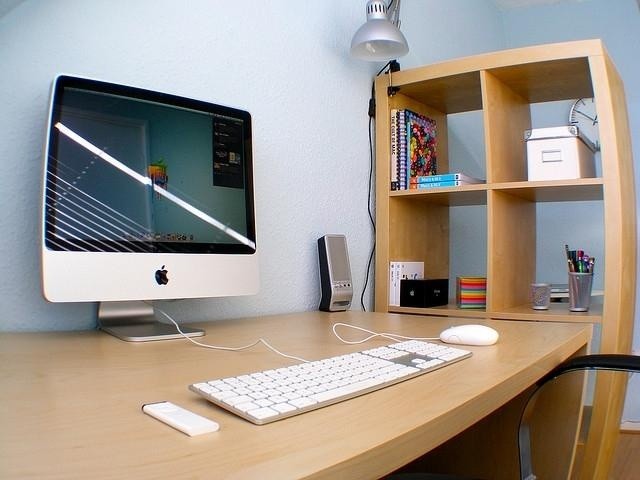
521;124;596;179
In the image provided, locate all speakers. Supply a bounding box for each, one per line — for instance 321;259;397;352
400;275;449;308
318;234;353;312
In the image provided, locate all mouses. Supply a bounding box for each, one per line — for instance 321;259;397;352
440;324;499;345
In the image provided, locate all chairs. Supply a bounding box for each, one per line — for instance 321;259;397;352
516;353;640;479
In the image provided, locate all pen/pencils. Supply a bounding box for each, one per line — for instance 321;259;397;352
565;245;595;308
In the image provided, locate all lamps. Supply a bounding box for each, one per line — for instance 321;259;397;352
349;0;409;73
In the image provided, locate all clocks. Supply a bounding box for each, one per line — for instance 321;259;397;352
568;94;600;150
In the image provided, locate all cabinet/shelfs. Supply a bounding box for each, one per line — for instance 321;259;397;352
376;38;640;479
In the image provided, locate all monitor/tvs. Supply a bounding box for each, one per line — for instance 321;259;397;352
42;74;259;342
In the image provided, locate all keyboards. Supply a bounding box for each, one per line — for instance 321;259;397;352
189;339;473;425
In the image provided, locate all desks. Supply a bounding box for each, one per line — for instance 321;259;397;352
0;307;594;480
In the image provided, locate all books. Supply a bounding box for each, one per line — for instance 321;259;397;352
389;107;486;192
388;258;424;306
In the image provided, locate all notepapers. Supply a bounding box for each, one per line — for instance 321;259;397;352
455;276;487;309
